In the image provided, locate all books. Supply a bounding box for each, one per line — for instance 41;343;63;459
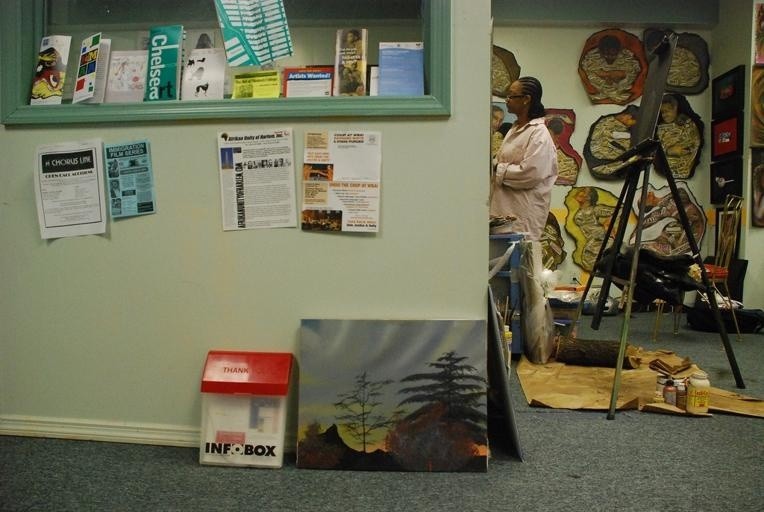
376;42;424;95
69;23;226;105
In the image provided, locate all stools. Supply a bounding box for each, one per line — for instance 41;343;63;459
652;264;743;344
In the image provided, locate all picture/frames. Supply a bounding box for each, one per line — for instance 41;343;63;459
710;156;744;205
712;63;746;120
715;207;742;260
711;110;744;162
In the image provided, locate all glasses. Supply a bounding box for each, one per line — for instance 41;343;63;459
507;95;524;99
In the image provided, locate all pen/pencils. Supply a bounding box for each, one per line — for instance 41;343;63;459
496;296;519;327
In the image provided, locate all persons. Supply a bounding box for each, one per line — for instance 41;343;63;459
656;97;700;179
631;188;703;258
589;103;640;174
572;188;620;267
490;104;505;159
343;30;361;58
346;62;360;81
583;35;642;104
491;77;557;242
